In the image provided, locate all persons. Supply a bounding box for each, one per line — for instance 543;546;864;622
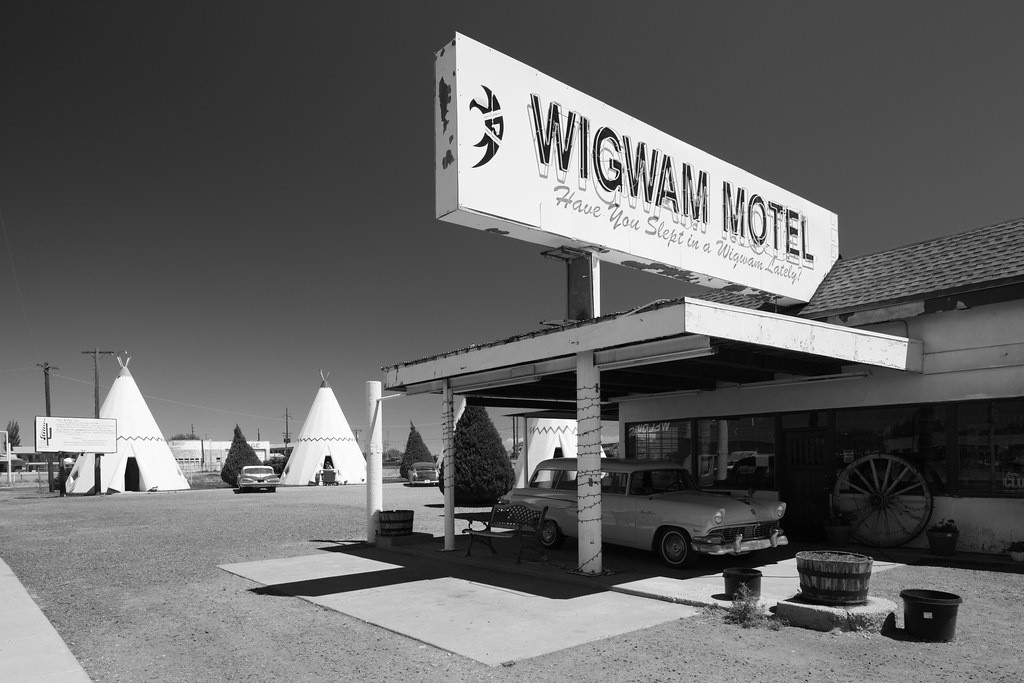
640;475;655;494
325;461;332;468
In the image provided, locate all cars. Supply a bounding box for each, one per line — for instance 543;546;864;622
236;465;281;493
408;462;440;486
498;457;790;568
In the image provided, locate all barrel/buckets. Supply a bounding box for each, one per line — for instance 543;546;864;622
795;550;873;605
723;568;762;600
900;589;963;643
379;510;414;535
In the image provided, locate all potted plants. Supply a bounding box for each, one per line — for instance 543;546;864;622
926;518;961;555
823;507;855;547
795;550;873;606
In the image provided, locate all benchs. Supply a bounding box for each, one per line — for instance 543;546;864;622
461;501;550;565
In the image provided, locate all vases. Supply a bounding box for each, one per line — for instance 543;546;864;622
379;511;416;536
898;586;964;644
723;568;764;600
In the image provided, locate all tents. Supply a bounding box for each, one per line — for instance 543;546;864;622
434;398;467;480
510;417;611;490
64;356;192;494
280;370;367;485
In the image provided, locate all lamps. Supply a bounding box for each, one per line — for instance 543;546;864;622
737;371;866;389
453;374;543;394
596;346;718;372
608;389;701;402
